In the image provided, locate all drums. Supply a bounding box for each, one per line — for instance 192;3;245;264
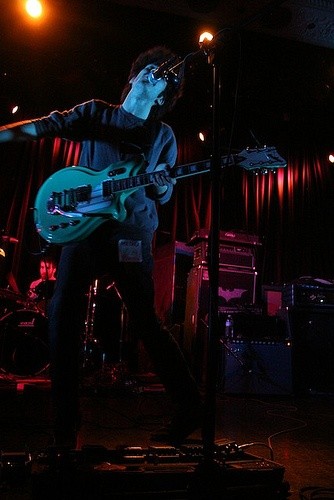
0;309;52;379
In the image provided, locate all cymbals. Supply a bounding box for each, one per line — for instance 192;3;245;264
0;235;19;244
1;286;26;302
34;279;57;298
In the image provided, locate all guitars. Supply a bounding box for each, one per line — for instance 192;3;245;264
33;146;288;247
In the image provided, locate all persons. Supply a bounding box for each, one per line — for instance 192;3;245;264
0;46;203;465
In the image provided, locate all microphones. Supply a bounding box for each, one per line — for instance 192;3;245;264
152;56;176;80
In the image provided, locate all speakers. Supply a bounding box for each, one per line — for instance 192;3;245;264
221;338;292;396
272;306;334;396
185;265;257;388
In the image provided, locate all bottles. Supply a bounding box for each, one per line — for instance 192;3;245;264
225;315;234;337
